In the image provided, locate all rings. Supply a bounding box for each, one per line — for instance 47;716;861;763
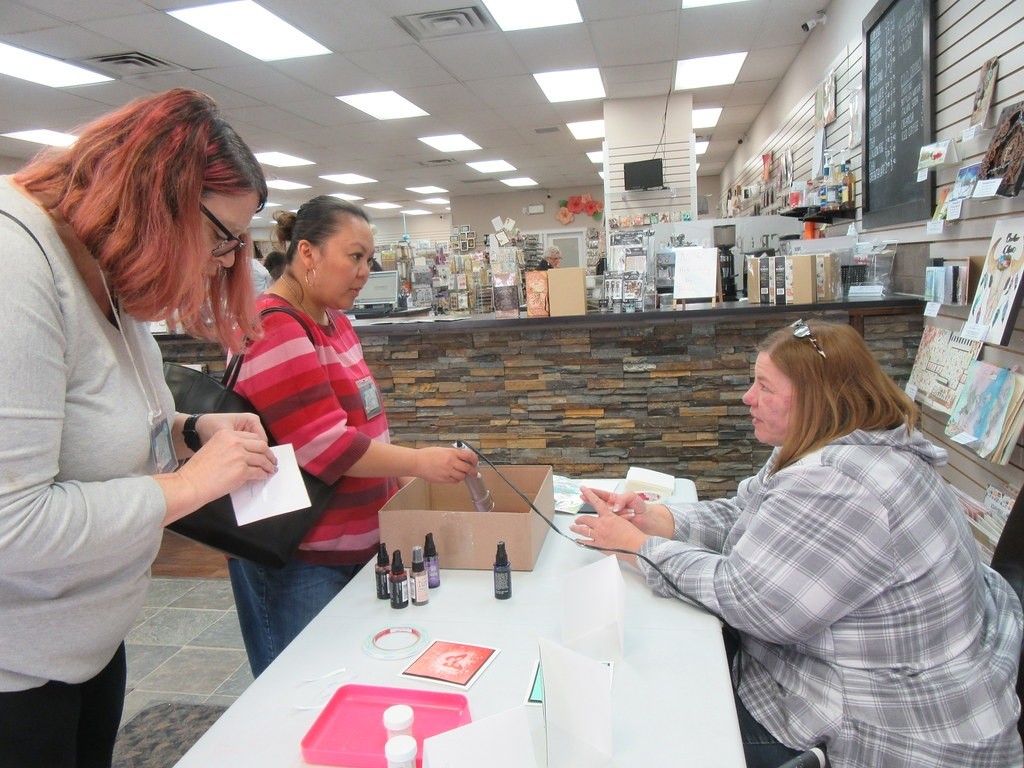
588;527;592;537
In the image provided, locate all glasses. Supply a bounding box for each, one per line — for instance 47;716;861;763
198;201;245;258
785;318;827;360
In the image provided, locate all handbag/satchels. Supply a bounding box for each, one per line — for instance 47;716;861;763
162;306;341;569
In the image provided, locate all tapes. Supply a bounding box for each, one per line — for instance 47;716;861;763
366;624;430;660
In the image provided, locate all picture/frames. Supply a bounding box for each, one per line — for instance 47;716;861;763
450;225;476;251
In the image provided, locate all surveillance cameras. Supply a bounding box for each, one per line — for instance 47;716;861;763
802;20;816;32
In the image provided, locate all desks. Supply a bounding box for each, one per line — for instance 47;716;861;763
172;479;748;768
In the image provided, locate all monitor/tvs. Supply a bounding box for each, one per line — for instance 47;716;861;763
624;158;663;191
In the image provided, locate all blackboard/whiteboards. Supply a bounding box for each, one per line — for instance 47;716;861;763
859;0;936;230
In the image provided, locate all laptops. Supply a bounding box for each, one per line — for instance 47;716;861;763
343;270;400;315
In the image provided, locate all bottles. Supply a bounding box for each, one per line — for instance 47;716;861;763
841;160;855;211
374;542;390;599
423;532;440;588
493;540;512;599
388;550;408;609
408;545;429;606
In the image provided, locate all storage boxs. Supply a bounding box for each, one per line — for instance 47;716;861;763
548;267;587;316
378;466;553;570
749;253;842;304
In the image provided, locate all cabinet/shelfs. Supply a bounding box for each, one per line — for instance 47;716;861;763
382;241;546;313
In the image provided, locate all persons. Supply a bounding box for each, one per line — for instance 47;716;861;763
0;88;278;768
244;243;288;297
536;245;562;271
573;318;1024;767
225;195;477;680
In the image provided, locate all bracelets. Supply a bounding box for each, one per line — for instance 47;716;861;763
182;413;204;454
